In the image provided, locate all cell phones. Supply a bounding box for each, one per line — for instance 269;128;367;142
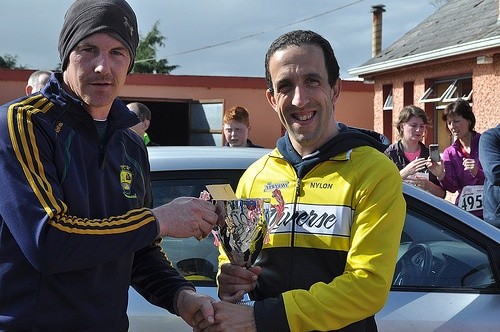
429;144;440;162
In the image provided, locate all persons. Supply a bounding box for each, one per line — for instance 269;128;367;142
383;105;446;200
0;0;219;332
125;103;161;146
191;30;406;332
222;106;266;148
426;99;484;221
479;123;500;230
25;70;53;96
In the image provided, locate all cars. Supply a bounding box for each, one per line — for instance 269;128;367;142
126;146;499;332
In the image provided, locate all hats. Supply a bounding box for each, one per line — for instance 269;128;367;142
57;0;141;76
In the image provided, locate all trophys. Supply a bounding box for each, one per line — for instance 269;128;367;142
205;197;270;303
